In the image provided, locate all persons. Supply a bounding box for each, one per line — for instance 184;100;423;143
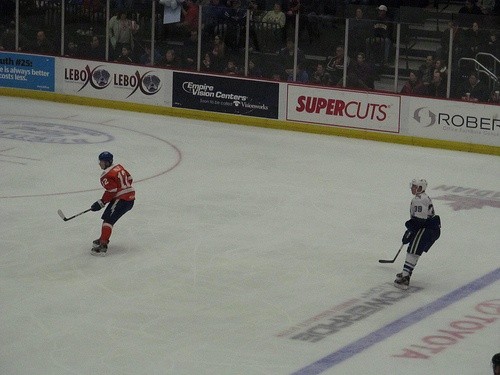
394;177;441;290
90;151;136;257
0;0;500;105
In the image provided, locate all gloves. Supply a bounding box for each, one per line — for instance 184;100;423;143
91;199;105;211
402;239;408;245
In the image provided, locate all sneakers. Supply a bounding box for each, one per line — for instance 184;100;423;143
396;273;403;279
92;239;109;248
90;245;107;257
394;276;409;289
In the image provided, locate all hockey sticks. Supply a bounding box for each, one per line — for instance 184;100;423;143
378;229;409;264
56;208;92;222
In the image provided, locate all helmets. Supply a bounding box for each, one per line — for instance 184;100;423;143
412;178;427;191
99;152;113;161
